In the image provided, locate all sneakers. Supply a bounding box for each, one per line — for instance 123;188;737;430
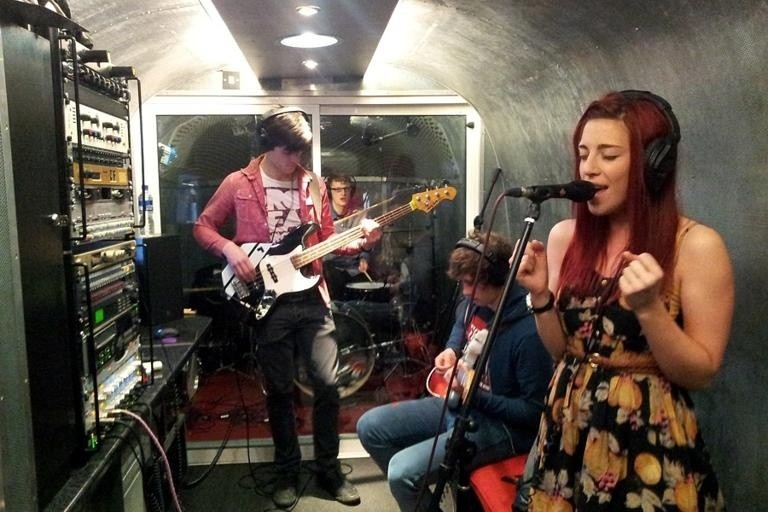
271;482;298;509
313;473;361;505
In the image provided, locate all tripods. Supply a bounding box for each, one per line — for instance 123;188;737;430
215;322;272;398
376;290;434;392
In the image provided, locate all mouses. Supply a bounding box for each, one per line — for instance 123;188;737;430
154;328;180;338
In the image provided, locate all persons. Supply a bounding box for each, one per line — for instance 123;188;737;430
357;232;555;511
191;106;384;507
323;174;382;276
508;88;738;512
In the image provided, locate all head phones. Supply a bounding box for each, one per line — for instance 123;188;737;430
256;107;310;147
324;170;357;199
622;87;682;194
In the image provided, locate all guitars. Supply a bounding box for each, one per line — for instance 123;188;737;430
219;179;456;318
445;328;489;412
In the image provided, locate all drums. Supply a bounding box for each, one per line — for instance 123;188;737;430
290;298;379;400
344;279;390;307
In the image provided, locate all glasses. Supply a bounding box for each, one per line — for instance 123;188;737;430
330;186;351;192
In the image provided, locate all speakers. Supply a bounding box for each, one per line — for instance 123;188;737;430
136;234;186;328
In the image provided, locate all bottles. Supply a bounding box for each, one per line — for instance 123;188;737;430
184;184;198;223
139;185;155;236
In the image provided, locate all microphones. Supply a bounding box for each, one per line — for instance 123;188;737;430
502;179;596;202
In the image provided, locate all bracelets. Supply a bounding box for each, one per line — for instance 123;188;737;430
526;288;555;314
